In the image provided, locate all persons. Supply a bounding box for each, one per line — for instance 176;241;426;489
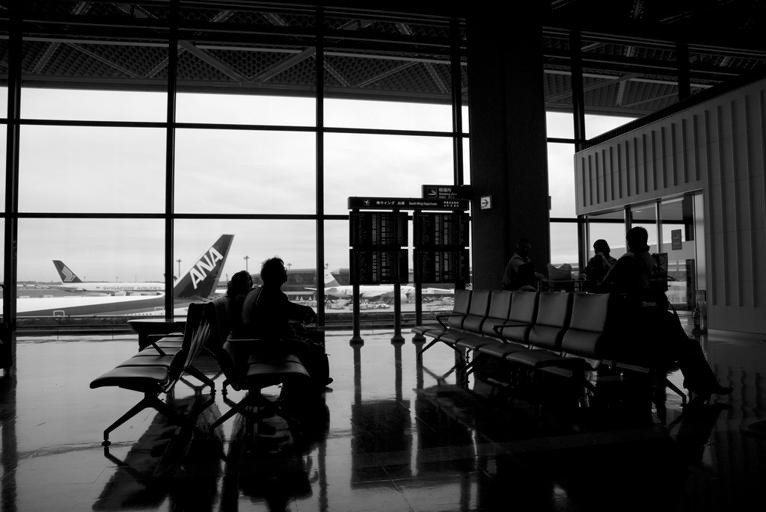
225;269;260;339
644;275;734;396
501;238;548;291
578;239;618;293
583;227;656;366
241;257;334;390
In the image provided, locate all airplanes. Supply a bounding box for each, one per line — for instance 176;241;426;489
16;259;166;297
304;267;454;305
1;233;236;326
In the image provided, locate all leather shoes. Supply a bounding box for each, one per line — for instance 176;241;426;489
652;389;666;401
715;384;733;395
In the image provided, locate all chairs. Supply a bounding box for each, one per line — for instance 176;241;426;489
411;289;688;414
88;303;312;444
94;393;318;508
